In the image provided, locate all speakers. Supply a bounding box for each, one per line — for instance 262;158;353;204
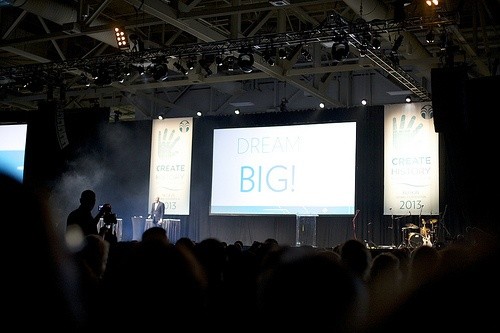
455;75;500;147
431;67;468;132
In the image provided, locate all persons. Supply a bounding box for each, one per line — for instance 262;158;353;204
66;190;110;239
0;172;500;333
152;197;164;227
